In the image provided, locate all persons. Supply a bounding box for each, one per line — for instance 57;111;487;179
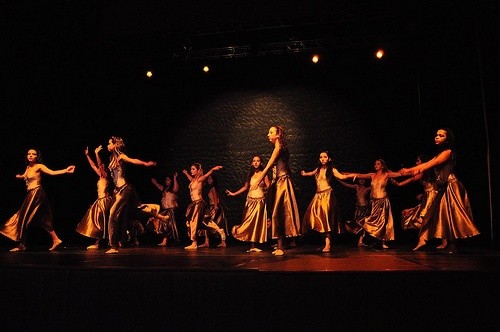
0;147;76;253
338;125;481;254
76;136;230;253
301;150;359;253
224;154;271;252
251;124;301;256
